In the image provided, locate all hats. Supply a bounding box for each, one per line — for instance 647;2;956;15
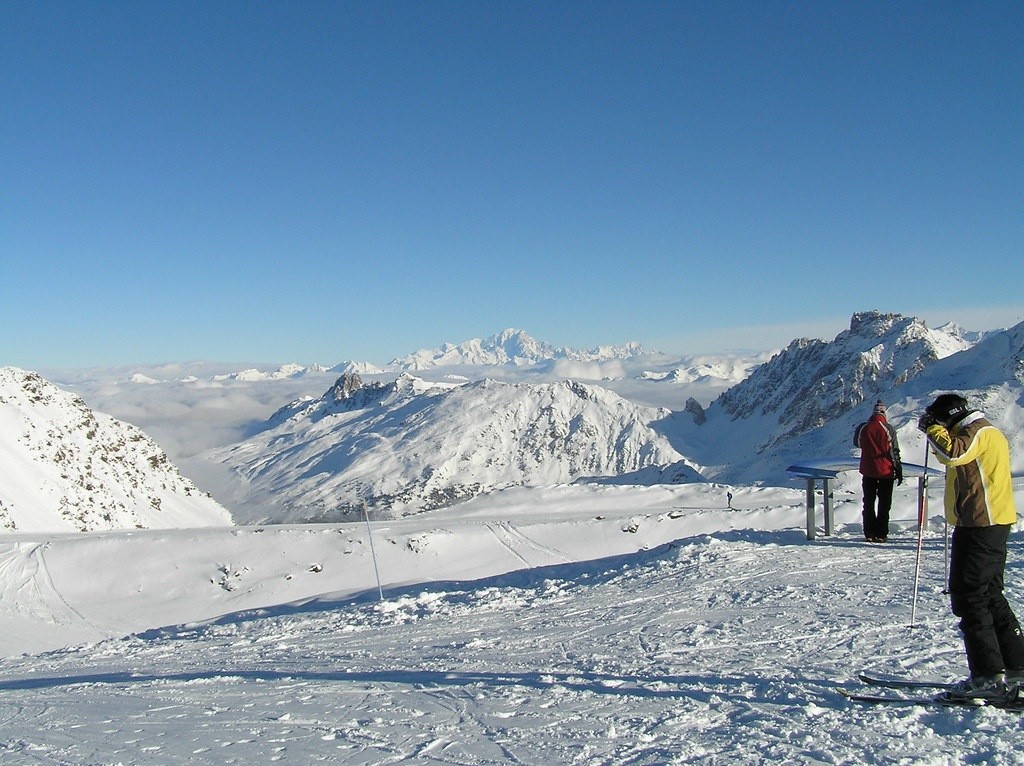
873;400;886;414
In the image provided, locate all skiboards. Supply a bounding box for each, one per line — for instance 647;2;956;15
836;674;1023;712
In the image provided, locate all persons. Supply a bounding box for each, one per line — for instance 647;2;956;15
853;399;903;543
918;393;1024;698
727;492;732;507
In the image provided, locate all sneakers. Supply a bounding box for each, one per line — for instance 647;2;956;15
934;669;1024;710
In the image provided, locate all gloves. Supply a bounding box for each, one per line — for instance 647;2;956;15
894;466;903;486
918;412;936;433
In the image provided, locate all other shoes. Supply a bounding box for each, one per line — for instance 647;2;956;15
865;535;887;543
